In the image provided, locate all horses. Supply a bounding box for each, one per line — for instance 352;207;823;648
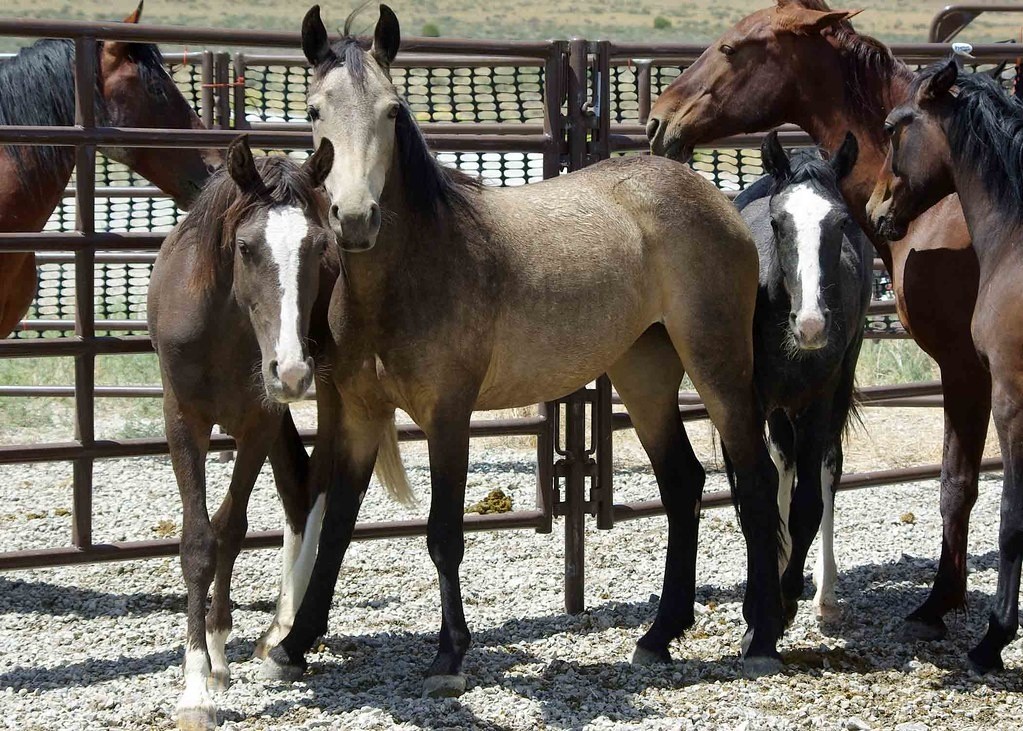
144;129;410;731
250;2;789;700
731;128;877;635
860;53;1023;679
644;0;994;644
0;0;228;342
